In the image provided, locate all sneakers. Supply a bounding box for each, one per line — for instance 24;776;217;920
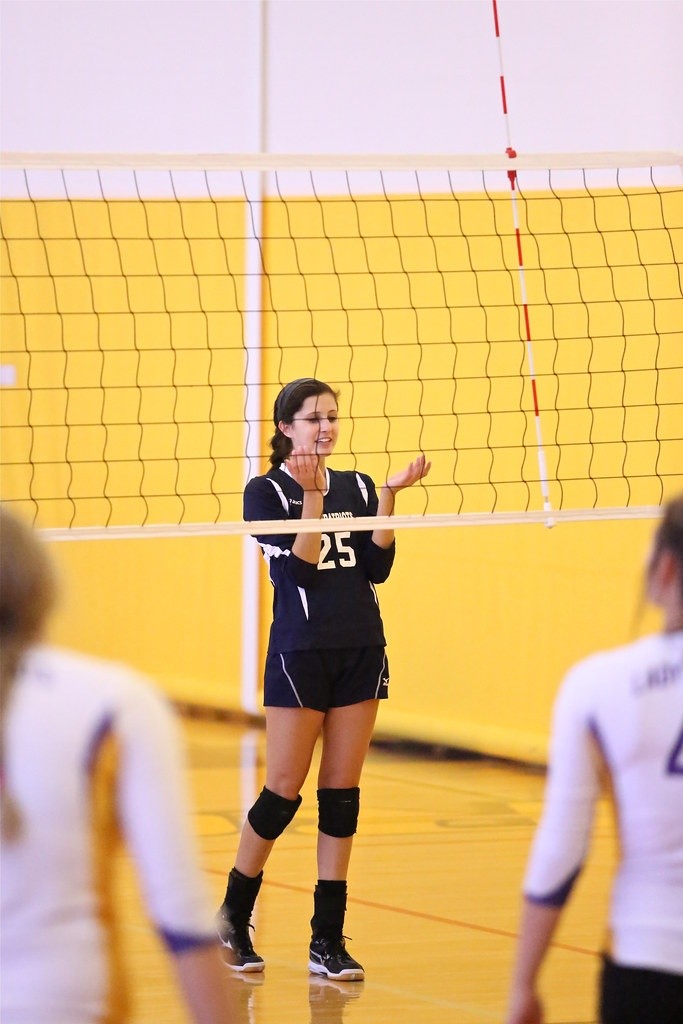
211;909;265;973
307;937;366;982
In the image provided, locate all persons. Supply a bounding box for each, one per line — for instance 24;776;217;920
507;489;683;1024
0;501;249;1024
210;376;433;981
